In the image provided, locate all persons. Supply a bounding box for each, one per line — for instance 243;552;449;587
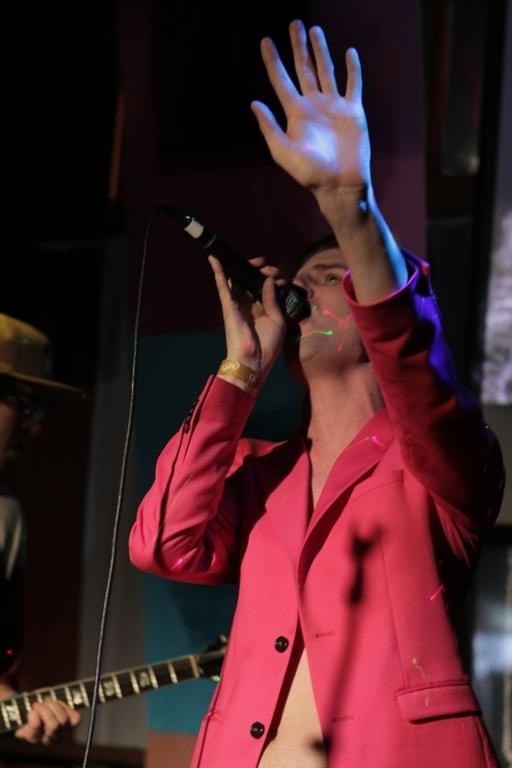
124;16;500;768
0;313;89;768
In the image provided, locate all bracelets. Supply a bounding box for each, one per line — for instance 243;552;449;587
212;359;268;394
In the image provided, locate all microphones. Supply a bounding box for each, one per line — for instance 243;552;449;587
174;209;311;323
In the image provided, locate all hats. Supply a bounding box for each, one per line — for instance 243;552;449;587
0;311;88;401
